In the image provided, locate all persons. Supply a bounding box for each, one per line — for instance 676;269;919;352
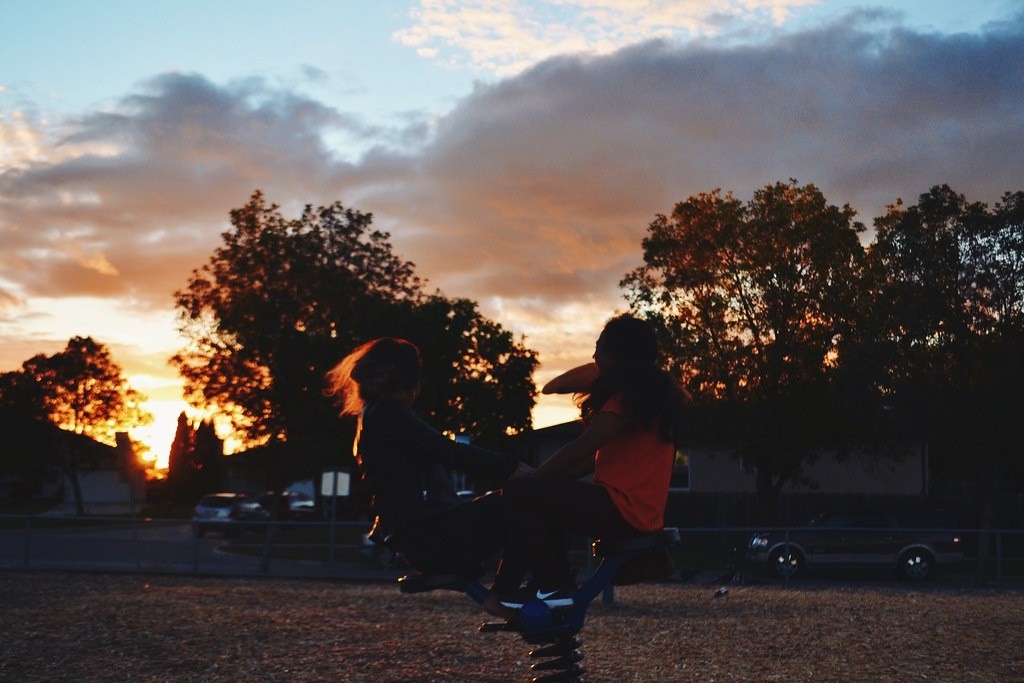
350;338;535;577
466;318;684;575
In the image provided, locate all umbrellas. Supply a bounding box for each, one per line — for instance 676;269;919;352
541;363;599;395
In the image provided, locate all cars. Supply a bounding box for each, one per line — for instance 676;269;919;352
192;492;273;539
729;500;964;583
256;489;322;524
